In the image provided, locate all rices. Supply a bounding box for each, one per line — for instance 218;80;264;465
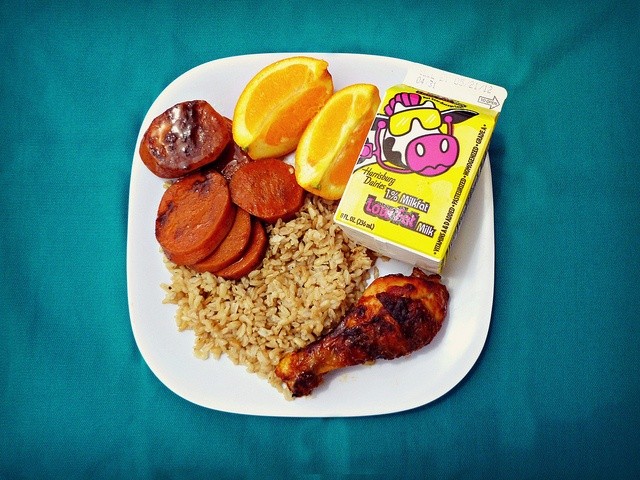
160;194;391;404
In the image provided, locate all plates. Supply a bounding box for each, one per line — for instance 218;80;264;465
126;51;496;417
